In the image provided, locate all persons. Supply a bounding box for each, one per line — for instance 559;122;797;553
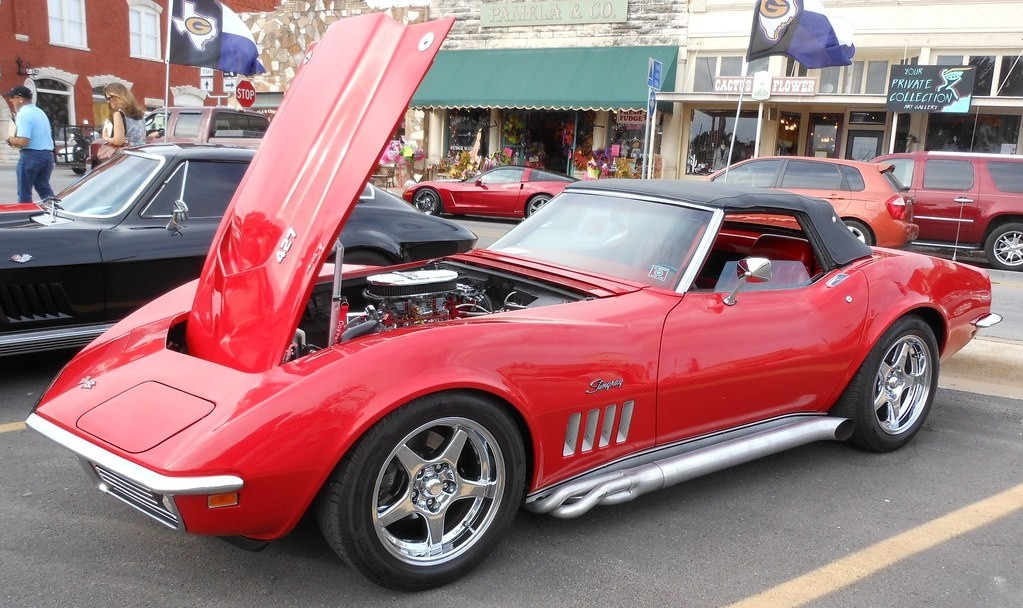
6;86;56;203
102;82;148;148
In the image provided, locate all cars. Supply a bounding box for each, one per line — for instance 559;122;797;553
400;167;580;222
704;157;921;250
91;105;270;171
0;142;479;360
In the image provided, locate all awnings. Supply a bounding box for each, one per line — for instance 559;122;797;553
408;45;679;114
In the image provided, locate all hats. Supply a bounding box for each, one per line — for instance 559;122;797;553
4;86;33;98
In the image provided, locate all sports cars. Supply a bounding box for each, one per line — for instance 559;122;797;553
24;16;1007;594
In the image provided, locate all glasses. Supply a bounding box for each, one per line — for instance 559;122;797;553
10;95;19;99
107;96;114;101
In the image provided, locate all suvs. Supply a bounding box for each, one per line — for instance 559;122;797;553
867;153;1023;273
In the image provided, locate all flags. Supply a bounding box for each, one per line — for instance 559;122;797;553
166;1;266;76
745;0;855;69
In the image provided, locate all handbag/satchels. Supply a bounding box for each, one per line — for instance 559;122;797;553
96;109;128;163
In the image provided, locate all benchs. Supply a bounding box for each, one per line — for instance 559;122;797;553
371;164;396;189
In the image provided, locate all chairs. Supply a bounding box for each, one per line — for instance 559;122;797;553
722;233;814;292
